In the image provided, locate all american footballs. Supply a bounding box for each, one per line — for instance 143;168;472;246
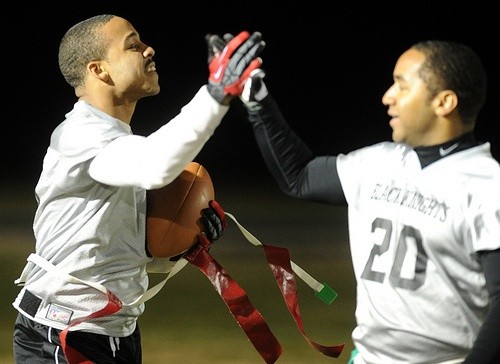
145;161;215;258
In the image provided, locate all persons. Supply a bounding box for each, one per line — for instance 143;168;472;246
11;15;267;364
202;30;500;364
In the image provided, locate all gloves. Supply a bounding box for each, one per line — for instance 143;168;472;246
198;200;229;251
207;32;266;105
204;29;271;109
